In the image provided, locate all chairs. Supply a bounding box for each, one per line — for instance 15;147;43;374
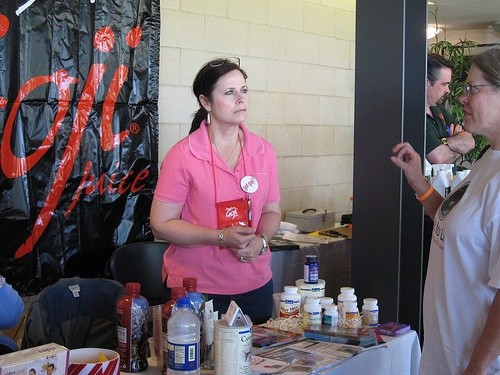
20;241;171;351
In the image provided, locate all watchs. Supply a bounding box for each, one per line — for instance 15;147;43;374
440;138;448;144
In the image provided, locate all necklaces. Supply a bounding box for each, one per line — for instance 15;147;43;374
211;136;238;163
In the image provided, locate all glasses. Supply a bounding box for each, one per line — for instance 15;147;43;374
464;84;498;95
200;56;240;94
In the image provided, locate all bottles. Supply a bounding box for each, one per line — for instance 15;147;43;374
338;287;357;313
362;298;378;328
347;196;353;214
323;305;339;327
179;277;208;364
304;254;319;284
280;286;302;318
166;297;201;375
116;283;148;373
303;297;322;325
341;301;359;329
170;296;199;319
320;298;333;318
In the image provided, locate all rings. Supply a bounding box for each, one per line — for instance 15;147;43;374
241;257;243;259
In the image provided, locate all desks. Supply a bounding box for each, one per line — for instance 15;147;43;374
121;323;422;375
269;221;353;304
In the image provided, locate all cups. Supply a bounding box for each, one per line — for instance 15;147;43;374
213;319;253;375
200;324;214;375
295;279;326;312
66;348;120;375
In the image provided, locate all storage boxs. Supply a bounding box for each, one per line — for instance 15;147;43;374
0;342;70;375
285;209;336;233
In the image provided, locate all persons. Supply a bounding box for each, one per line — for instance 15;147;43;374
47;364;54;375
29;369;36;375
424;54;476;272
150;57;281;327
390;47;500;375
0;275;25;357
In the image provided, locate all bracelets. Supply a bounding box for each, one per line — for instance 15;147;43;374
219;228;224;247
260;236;268;254
415;184;433;202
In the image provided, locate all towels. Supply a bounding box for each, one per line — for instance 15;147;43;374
276;222;299;236
